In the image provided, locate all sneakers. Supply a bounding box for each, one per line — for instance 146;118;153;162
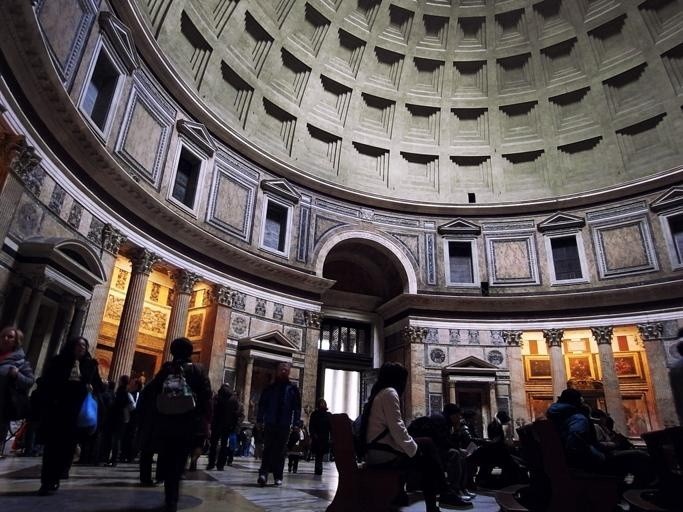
428;482;480;512
274;478;282;485
257;474;266;485
158;506;176;512
154;479;164;487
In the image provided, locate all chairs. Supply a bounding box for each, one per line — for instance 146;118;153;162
327;414;619;511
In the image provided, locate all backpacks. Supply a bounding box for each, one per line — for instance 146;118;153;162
351;384;392;460
157;365;197;414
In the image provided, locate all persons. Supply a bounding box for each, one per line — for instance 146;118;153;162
1;328;683;511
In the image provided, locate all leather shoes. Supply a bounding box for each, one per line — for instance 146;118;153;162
39;483;49;496
49;478;59;491
189;461;233;471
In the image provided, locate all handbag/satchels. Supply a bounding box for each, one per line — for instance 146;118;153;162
642;427;683;510
3;375;30;422
